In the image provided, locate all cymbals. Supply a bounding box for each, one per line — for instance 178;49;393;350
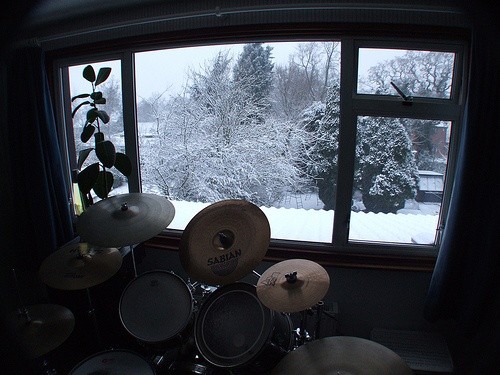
39;242;123;290
76;193;175;248
270;336;410;375
255;259;331;314
179;199;271;287
0;303;75;364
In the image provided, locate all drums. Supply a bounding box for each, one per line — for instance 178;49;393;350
67;348;158;375
194;281;293;373
118;269;197;351
